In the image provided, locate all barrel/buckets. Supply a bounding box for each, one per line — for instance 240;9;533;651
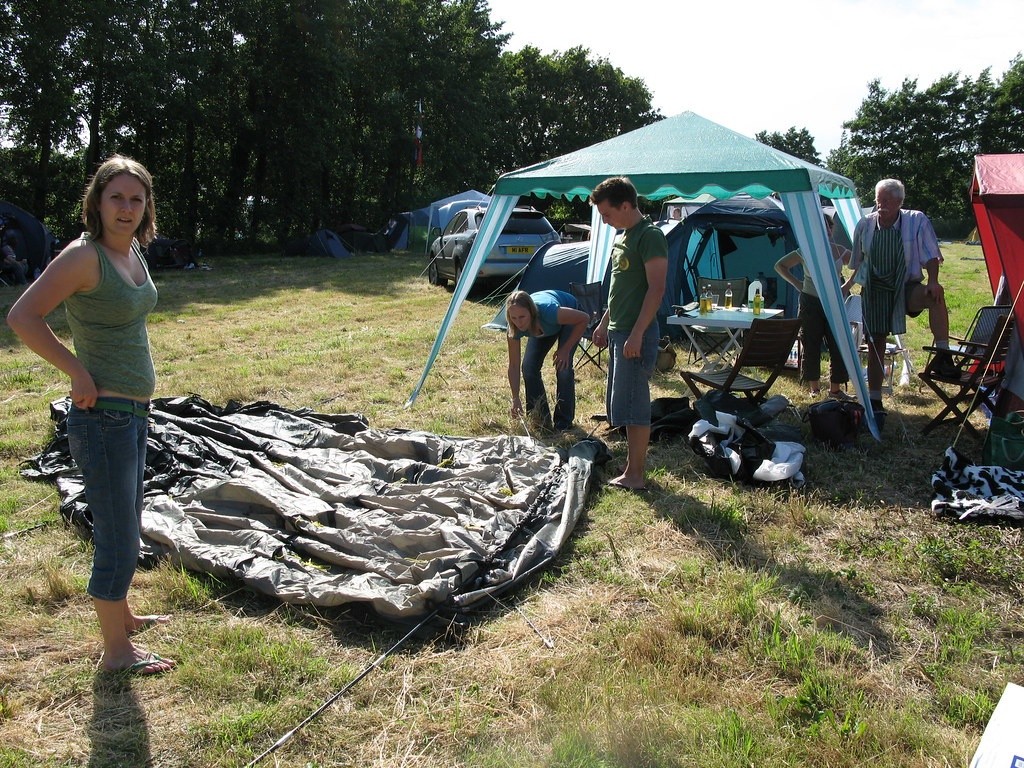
991;430;1024;472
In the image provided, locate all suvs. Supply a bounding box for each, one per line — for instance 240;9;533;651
427;205;562;300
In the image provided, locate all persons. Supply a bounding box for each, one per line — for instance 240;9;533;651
847;177;963;419
773;213;854;403
591;175;670;492
5;153;176;677
504;288;591;434
0;222;29;285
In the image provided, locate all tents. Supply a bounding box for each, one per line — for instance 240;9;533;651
489;192;811;345
139;233;197;272
0;199;59;281
283;188;490;259
404;106;883;443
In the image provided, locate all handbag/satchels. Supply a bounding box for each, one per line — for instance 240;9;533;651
802;400;865;443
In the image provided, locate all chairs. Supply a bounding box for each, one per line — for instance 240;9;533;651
568;281;610;376
680;315;804;404
845;294;905;395
918;305;1014;447
687;276;748;366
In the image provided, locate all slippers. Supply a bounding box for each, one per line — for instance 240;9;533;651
606;479;647;490
98;652;178;677
127;613;172;637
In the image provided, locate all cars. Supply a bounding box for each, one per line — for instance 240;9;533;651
655;199;709;227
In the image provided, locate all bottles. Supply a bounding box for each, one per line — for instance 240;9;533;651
753;287;760;315
700;286;707;316
725;282;732;309
748;278;764;313
706;284;712;312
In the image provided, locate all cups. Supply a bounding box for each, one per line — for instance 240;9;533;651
741;303;748;312
712;295;720;311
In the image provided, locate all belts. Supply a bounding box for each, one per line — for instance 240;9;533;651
94;400;150;419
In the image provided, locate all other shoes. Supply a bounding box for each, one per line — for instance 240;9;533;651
869;398;887;431
933;354;961;376
809;390;822;398
828;390;858;400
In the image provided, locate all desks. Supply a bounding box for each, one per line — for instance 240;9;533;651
666;305;784;399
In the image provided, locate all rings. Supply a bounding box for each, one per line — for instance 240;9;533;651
560;360;563;362
631;354;636;355
597;335;601;339
